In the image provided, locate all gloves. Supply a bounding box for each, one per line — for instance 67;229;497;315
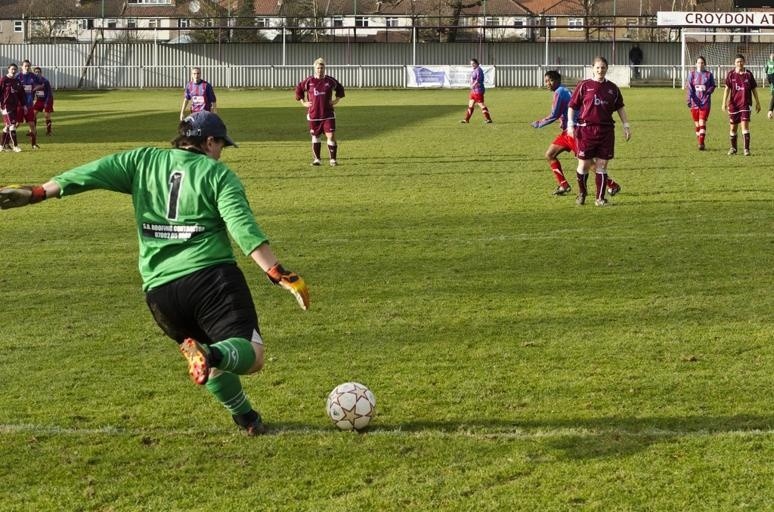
0;183;45;210
266;263;310;310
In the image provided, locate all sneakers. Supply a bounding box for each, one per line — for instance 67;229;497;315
310;160;337;166
26;130;37;136
47;131;53;136
246;413;265;436
485;120;492;123
179;337;210;385
460;120;468;123
0;144;39;152
554;184;620;206
699;145;750;155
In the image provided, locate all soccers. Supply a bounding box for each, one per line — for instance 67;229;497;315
327;382;377;430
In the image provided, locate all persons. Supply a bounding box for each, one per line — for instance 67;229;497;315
567;56;631;206
722;54;761;156
768;88;774;120
0;60;54;152
687;56;716;150
530;71;621;196
180;67;216;122
764;54;774;95
460;59;492;123
630;43;643;74
0;112;309;437
295;58;345;167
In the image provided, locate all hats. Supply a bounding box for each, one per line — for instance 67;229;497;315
179;111;238;149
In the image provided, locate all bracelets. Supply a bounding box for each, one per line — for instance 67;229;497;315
567;121;573;126
623;122;630;129
